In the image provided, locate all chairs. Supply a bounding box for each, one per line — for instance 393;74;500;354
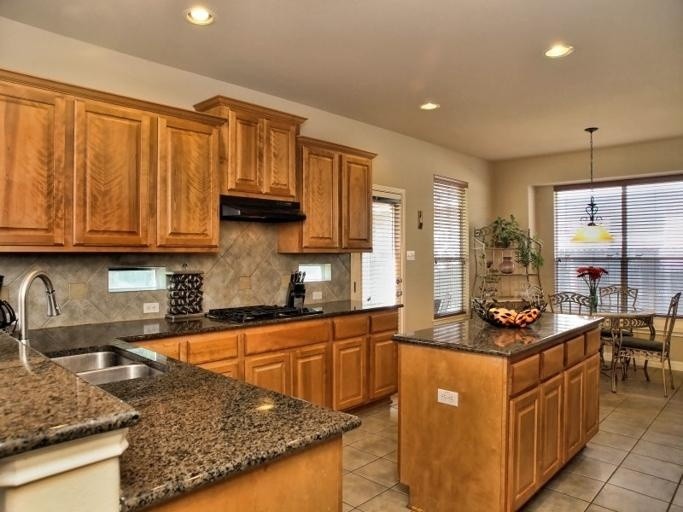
598;283;640;374
611;289;682;399
547;290;611;378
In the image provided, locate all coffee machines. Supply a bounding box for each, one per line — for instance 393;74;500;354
0;273;17;336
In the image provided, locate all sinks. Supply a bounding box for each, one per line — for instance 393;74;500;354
49;352;136;370
77;365;166;386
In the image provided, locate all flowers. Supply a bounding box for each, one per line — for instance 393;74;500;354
575;265;609;306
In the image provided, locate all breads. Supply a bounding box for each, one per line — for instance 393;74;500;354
515;308;539;325
488;307;517;326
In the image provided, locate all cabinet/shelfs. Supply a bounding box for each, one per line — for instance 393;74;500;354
129;329;242;381
397;343;563;512
561;326;602;463
0;67;67;254
67;85;227;254
193;96;308;203
471;216;548;329
331;310;399;411
240;317;333;409
275;134;377;256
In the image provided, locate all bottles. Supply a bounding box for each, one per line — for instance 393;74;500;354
498;256;516;273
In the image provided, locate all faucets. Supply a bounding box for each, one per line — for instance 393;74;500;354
18;342;37;376
18;271;59;340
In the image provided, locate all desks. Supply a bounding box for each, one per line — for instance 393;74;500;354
558;309;659;394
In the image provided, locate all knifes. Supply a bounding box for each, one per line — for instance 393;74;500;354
289;270;305;283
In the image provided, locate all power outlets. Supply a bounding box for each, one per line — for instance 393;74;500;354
436;387;459;409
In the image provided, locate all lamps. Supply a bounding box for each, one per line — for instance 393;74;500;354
565;125;615;245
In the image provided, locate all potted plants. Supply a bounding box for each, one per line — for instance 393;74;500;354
488;216;546;268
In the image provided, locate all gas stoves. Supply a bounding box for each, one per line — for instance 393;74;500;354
204;302;324;326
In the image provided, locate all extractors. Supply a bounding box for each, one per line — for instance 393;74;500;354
219;194;307;224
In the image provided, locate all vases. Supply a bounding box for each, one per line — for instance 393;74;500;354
587;285;598;311
497;256;514;275
